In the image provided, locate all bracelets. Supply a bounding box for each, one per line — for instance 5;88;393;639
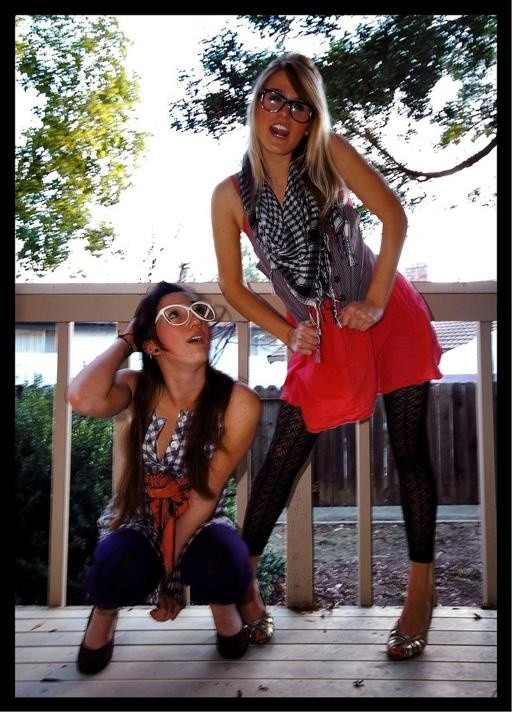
118;331;134;356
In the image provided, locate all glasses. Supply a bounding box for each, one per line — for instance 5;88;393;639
257;88;315;123
155;301;216;326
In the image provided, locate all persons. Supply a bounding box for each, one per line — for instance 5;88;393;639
212;54;442;661
67;281;263;674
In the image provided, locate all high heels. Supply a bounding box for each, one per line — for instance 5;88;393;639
78;606;115;675
386;584;433;660
216;578;275;660
